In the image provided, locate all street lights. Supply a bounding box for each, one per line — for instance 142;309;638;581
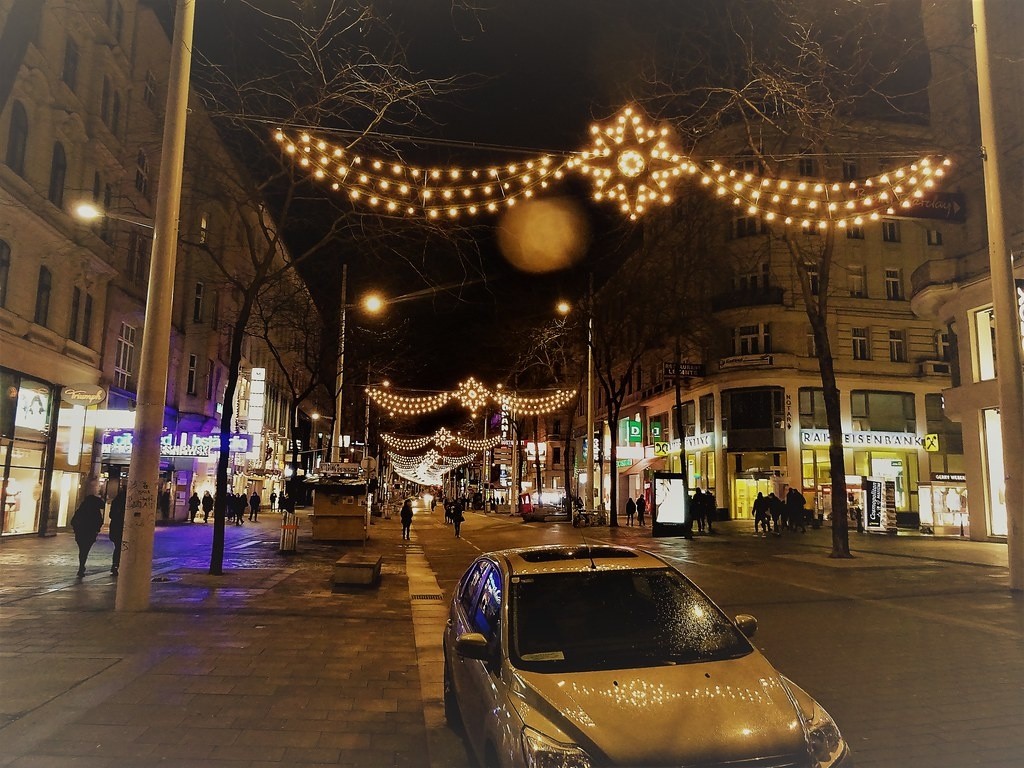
305;262;387;461
471;408;495;509
374;411;394;479
350;361;390;475
555;297;609;516
72;0;204;610
495;372;531;517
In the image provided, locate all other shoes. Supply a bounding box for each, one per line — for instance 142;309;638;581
203;518;207;523
631;524;633;527
455;534;460;537
762;528;766;531
708;525;712;531
111;566;119;576
191;521;195;523
698;528;705;532
77;567;86;577
235;521;239;523
236;523;239;526
241;521;244;525
755;530;758;532
639;524;641;526
626;524;629;526
403;537;410;540
644;523;645;526
768;528;772;532
445;521;452;524
248;518;251;521
227;519;232;522
800;529;806;533
233;521;235;522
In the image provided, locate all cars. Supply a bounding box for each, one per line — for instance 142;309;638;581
440;540;856;768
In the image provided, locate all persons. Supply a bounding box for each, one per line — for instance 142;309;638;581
688;487;717;533
626;498;636;527
636;494;645;526
489;496;504;507
431;495;467;538
70;484;128;576
159;492;170;526
270;491;284;514
574;513;589;528
401;499;414;541
578;497;584;506
188;490;261;526
752;487;808;537
